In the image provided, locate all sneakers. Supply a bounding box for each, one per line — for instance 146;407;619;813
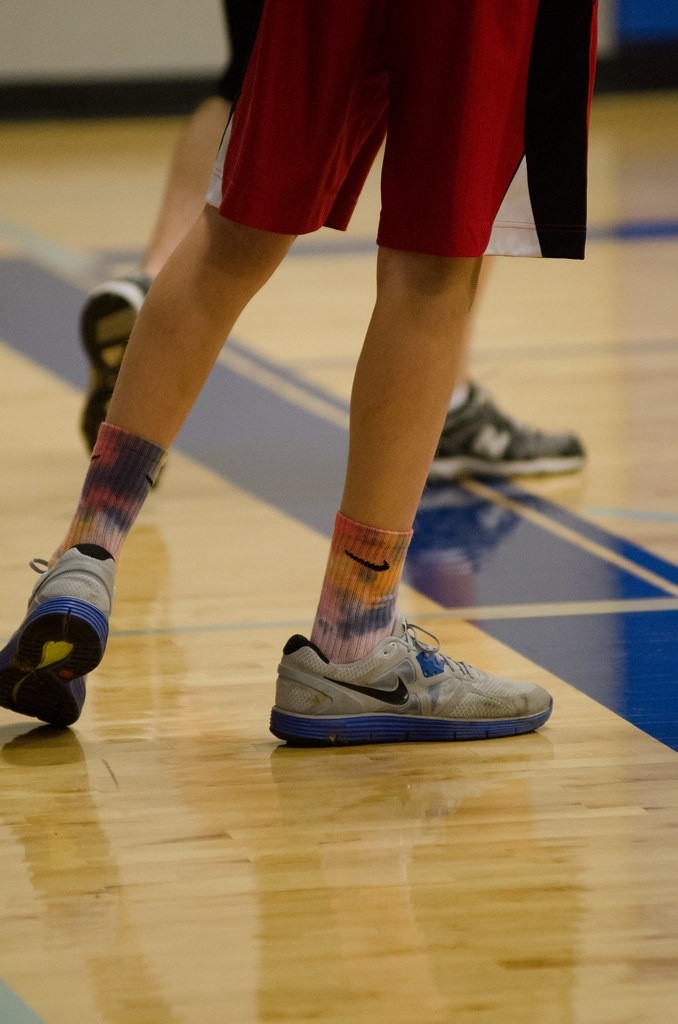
407;482;540;562
270;628;553;743
0;548;113;728
425;383;588;478
78;277;164;491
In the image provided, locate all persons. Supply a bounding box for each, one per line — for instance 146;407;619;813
0;0;599;743
79;0;587;476
